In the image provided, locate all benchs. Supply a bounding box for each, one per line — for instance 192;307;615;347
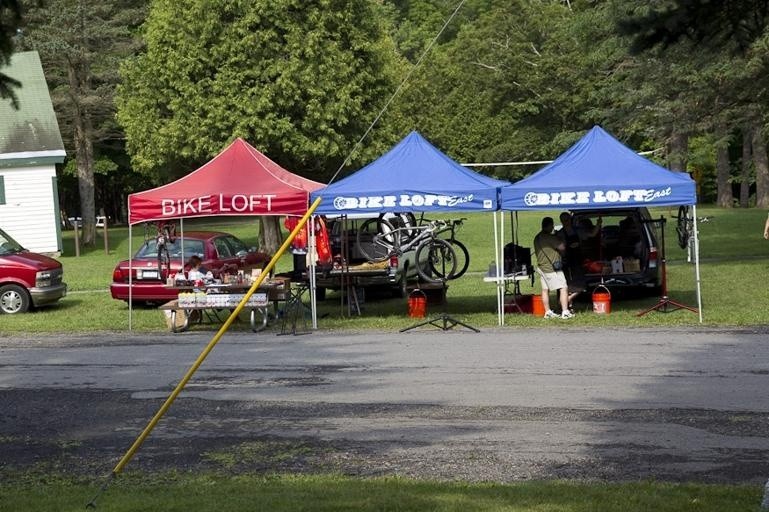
159;262;293;331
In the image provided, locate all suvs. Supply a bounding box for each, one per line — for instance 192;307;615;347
555;205;668;301
293;210;435;298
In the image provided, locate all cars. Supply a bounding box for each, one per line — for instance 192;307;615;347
0;227;69;312
110;231;278;313
57;213;112;230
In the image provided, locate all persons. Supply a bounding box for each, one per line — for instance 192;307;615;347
178;255;246;324
552;211;603;314
763;209;769;240
534;215;575;321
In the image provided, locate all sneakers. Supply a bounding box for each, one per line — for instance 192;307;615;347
560;309;576;320
543;309;560;320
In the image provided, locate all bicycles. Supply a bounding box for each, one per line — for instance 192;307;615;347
354;203;473;287
673;201;715;270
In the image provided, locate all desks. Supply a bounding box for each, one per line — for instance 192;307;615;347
482;267;528;318
301;266;391;318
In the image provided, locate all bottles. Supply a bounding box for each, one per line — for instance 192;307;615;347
489;257;528;278
176;292;267;307
219;270;257;284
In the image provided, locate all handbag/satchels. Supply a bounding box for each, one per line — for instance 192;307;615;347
552;261;563;272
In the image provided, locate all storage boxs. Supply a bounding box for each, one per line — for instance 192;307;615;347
623;257;641;272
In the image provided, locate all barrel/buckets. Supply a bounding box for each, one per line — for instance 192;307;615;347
592;284;612;315
408;288;427;318
532;294;545;316
